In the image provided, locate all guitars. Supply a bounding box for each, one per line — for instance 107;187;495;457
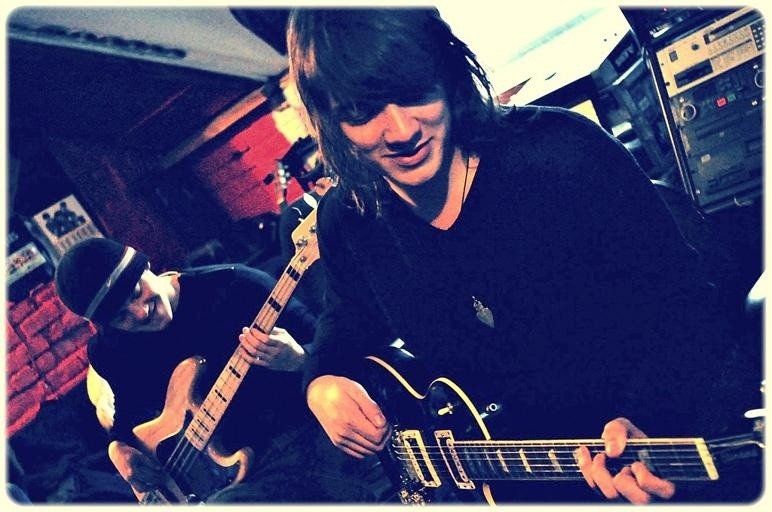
319;346;764;504
108;207;322;506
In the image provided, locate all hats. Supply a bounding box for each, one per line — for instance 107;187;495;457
55;238;148;325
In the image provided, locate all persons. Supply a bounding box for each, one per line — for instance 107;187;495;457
285;11;730;502
55;237;369;503
41;201;88;240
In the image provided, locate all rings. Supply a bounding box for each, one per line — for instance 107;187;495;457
256;351;265;361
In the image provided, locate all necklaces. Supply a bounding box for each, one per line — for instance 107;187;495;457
378;147;496;329
